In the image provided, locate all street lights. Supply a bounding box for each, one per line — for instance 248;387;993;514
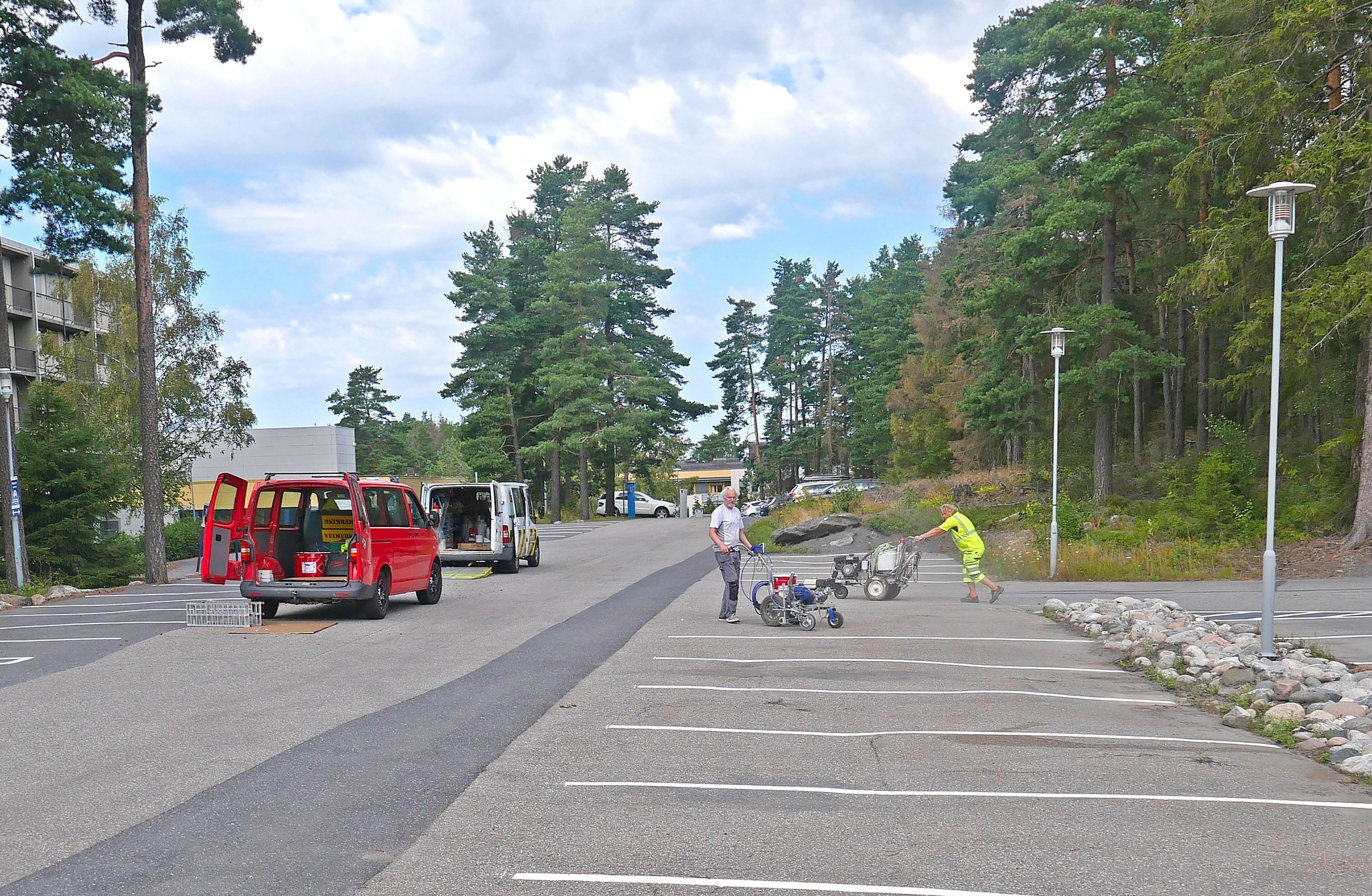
1244;181;1318;659
1039;326;1077;577
0;367;27;590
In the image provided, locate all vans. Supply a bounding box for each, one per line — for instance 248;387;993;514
419;479;541;574
200;471;442;619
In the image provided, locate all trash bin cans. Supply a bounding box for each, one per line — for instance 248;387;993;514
760;507;769;516
538;506;544;519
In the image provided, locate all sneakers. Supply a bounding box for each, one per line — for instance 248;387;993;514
960;593;979;603
989;586;1005;603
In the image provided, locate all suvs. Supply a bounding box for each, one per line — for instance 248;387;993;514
594;490;676;519
740;497;784;518
788;475;887;503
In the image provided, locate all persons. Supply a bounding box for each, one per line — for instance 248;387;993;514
914;504;1004;604
709;486;753;623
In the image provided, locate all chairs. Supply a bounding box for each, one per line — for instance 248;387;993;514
478;500;491;525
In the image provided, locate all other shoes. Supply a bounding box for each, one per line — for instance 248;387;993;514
719;614;741;623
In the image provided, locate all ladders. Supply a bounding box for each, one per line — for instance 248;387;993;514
186;601;265;628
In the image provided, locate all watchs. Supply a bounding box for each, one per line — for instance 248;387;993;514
920;534;925;540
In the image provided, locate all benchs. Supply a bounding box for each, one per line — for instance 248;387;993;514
302;510;353;552
